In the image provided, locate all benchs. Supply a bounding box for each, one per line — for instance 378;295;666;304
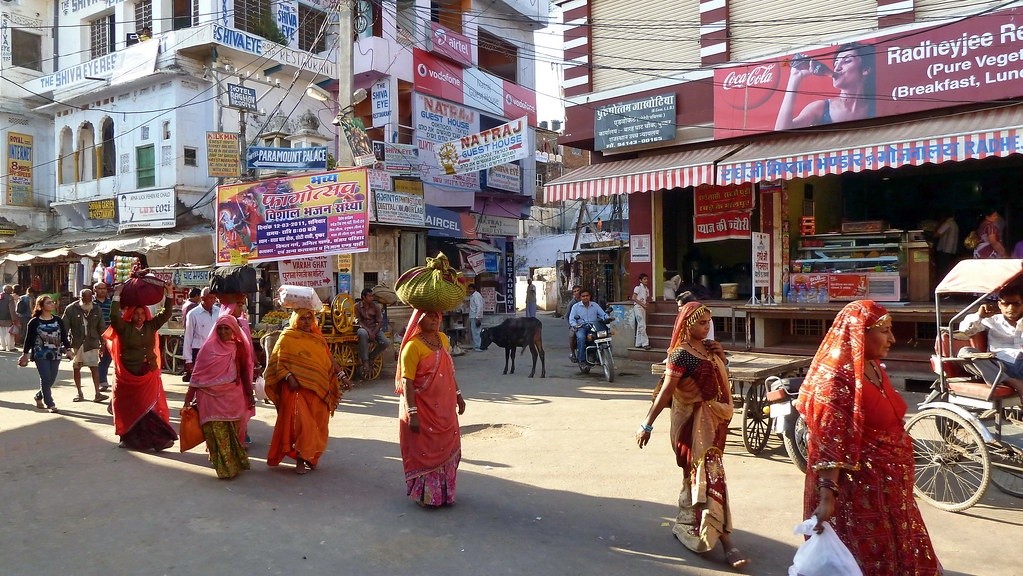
939;327;1014;402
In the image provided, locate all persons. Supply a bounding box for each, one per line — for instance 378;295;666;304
0;268;656;510
955;280;1023;406
637;302;751;568
119;195;135;223
925;201;1008;278
773;39;876;131
139;27;153;41
794;298;947;575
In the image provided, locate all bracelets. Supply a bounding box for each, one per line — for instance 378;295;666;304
725;358;730;367
818;477;839;499
640;422;654;434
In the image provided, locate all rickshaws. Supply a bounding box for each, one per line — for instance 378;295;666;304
651;348;816;456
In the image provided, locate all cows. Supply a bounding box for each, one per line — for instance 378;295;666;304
479;316;546;378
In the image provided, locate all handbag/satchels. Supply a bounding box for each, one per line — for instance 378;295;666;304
255;375;268;400
8;324;19;336
180;406;206;453
788;516;864;576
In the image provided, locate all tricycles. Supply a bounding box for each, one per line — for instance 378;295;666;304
899;257;1022;513
250;295;385;386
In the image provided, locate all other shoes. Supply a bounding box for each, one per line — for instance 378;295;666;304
34;385;109;413
296;467;307;473
644;346;651;350
725;547;747;569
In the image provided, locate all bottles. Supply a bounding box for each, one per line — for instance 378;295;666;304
817;287;829;304
798;284;807;304
787;285;798;304
789;54;837;79
806;286;817;304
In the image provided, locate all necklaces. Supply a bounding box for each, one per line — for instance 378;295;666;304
862;360;889;400
683;339;711;361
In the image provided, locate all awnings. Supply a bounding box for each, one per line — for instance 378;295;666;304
0;232;217;275
543;98;1022;207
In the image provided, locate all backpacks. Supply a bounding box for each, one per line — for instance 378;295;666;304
16;296;27;314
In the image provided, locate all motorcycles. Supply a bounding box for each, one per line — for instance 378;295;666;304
765;371;813;474
571;306;616;382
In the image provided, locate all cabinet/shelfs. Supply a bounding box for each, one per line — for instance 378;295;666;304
906;242;937;301
789;230;908;302
